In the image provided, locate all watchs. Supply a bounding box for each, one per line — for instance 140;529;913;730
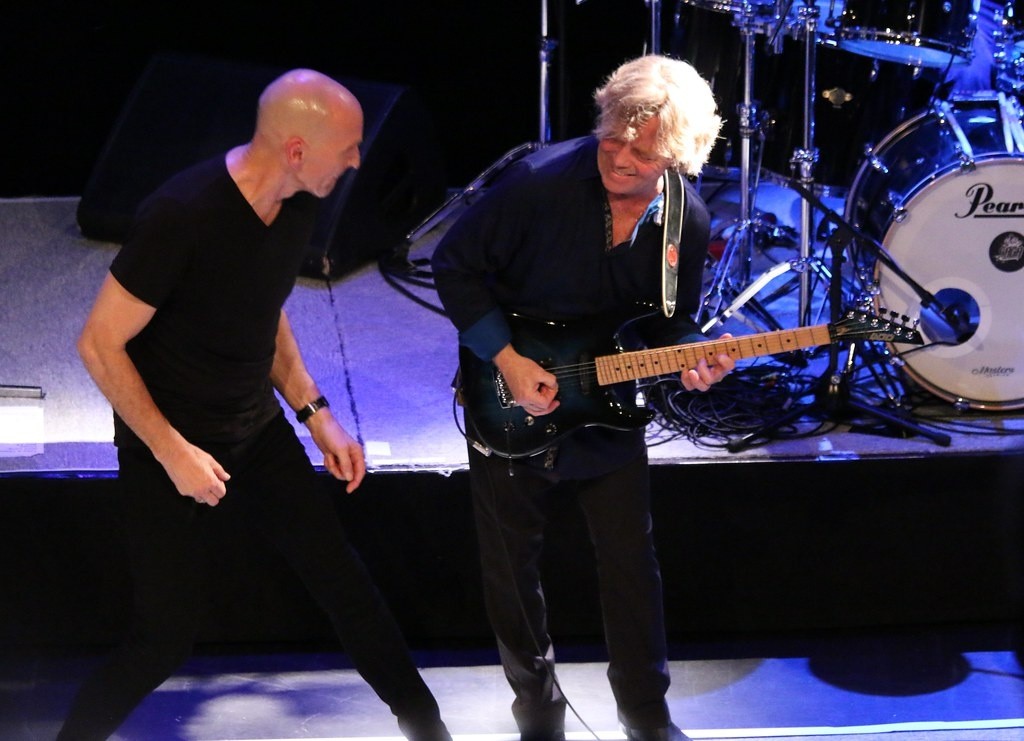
297;395;331;424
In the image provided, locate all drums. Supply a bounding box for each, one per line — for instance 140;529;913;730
835;0;980;69
752;27;975;200
734;0;846;45
685;0;775;15
843;87;1024;413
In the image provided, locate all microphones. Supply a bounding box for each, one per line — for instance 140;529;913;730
943;304;972;331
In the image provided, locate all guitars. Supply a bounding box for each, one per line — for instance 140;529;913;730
458;298;929;462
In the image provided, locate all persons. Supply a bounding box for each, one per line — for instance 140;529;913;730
430;55;735;741
56;68;454;741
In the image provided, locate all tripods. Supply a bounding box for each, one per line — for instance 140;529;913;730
690;0;953;450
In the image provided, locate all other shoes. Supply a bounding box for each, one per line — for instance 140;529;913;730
628;723;690;741
